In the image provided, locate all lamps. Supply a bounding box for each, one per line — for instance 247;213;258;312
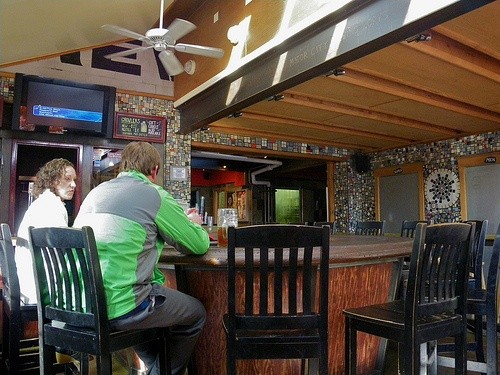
228;112;243;119
268;95;284;101
406;33;432;43
184;59;196;76
325;68;346;78
227;24;241;45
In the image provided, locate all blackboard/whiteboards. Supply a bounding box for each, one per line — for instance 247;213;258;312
113;111;167;144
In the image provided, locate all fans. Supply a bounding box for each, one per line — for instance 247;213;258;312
100;0;225;77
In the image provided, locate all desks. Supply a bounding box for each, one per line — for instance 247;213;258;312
112;233;413;375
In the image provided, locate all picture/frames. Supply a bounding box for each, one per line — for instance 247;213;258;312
112;111;166;143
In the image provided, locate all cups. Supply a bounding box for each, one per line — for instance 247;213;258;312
217;209;238;247
208;216;214;233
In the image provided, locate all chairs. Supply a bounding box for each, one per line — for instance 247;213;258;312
289;218;500;375
0;223;172;375
221;224;329;375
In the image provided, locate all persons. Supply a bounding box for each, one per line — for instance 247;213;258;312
15;158;77;363
50;142;211;375
